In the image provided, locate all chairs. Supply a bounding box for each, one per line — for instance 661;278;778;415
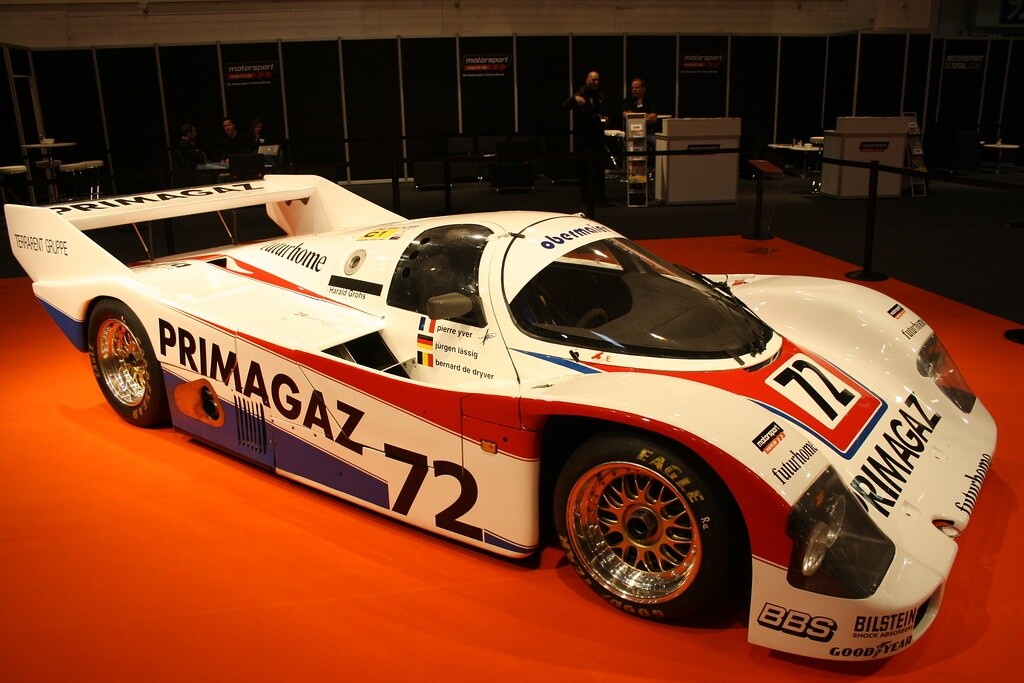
448;135;540;183
535;267;600;329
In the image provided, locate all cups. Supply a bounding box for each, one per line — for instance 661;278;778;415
804;143;813;149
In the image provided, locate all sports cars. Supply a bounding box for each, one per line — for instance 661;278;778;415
4;174;999;663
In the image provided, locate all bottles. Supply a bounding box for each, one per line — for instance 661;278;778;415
793;138;802;148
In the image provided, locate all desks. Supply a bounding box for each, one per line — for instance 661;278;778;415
768;143;823;180
21;142;76;204
983;144;1020;176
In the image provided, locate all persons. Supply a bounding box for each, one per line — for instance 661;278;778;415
565;70;615;207
623;78;657;165
171;118;266;188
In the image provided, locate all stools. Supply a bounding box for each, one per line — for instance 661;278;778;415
0;160;104;206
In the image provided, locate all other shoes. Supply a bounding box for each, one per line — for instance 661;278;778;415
592;198;616;207
